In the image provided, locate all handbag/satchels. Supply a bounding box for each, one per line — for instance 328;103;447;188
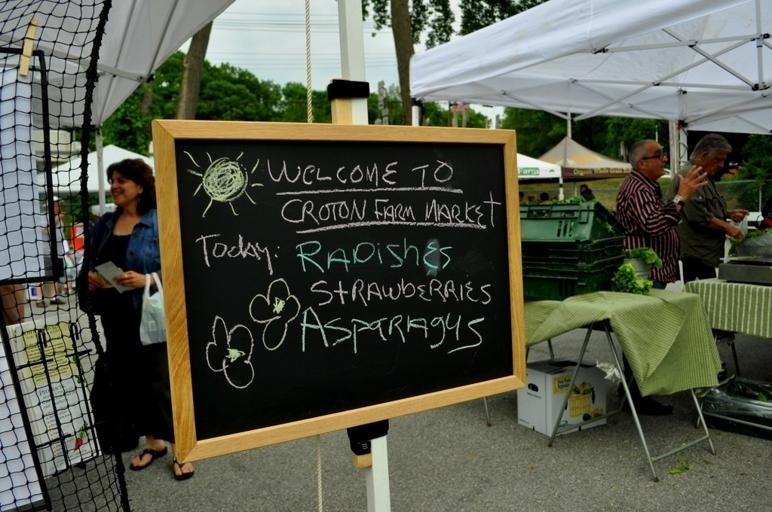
140;272;167;345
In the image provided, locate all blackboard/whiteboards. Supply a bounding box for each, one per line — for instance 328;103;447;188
150;120;528;466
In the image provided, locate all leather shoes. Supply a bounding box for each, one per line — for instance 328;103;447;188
620;394;673;415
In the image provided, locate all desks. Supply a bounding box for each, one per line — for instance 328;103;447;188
525;289;721;484
687;275;772;434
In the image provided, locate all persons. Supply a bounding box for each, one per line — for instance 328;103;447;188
580;184;594;201
77;156;194;482
539;192;554;205
519;192;528;205
36;195;66;309
60;228;77;296
663;133;751;287
525;195;539;206
616;139;709;417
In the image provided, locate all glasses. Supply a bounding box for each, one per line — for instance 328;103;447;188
642;150;667;160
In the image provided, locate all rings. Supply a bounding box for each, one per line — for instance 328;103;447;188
123;274;128;280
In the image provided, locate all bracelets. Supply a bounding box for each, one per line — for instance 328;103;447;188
673;193;686;207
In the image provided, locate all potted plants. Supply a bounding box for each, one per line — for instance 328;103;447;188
609;245;664;297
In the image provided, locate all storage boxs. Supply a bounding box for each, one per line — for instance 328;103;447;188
515;356;608;437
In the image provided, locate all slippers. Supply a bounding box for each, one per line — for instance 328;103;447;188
173;457;194;480
129;447;167;471
